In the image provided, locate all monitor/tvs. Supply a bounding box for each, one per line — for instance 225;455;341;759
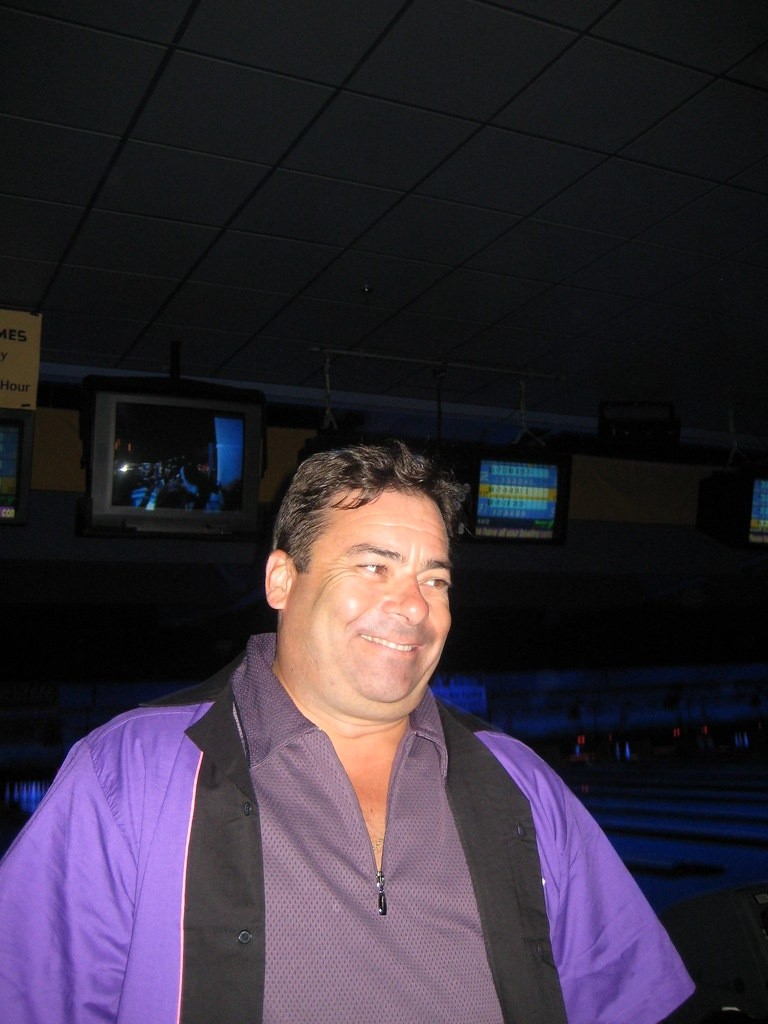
456;447;573;548
696;467;768;550
77;390;265;545
0;406;36;526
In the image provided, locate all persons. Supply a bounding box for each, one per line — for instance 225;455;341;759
0;439;697;1024
